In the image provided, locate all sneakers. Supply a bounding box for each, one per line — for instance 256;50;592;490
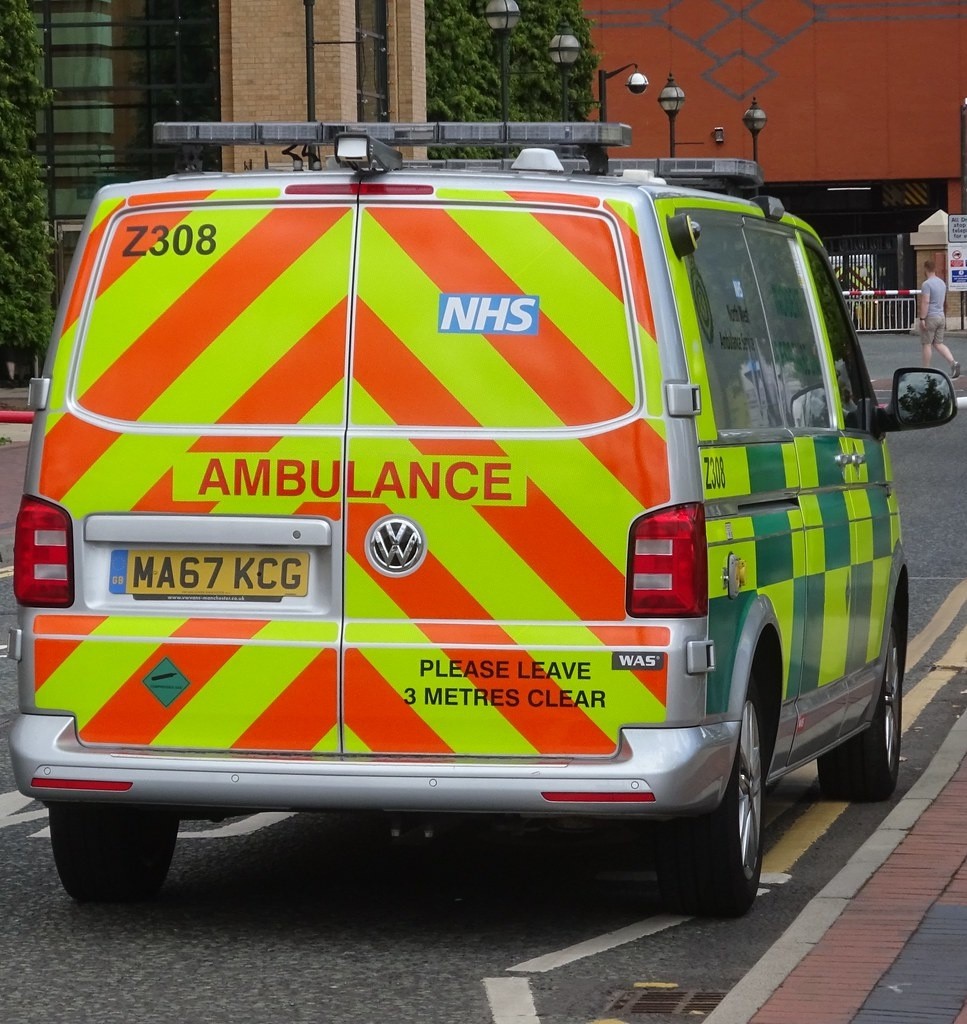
951;361;961;378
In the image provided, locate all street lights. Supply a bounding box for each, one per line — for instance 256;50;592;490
483;0;520;119
596;63;649;122
657;73;685;158
741;96;767;197
549;22;581;121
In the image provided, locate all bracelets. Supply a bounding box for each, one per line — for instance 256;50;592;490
920;318;925;321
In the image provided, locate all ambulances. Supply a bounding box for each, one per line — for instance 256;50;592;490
10;119;957;919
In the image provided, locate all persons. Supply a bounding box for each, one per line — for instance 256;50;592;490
920;260;960;381
